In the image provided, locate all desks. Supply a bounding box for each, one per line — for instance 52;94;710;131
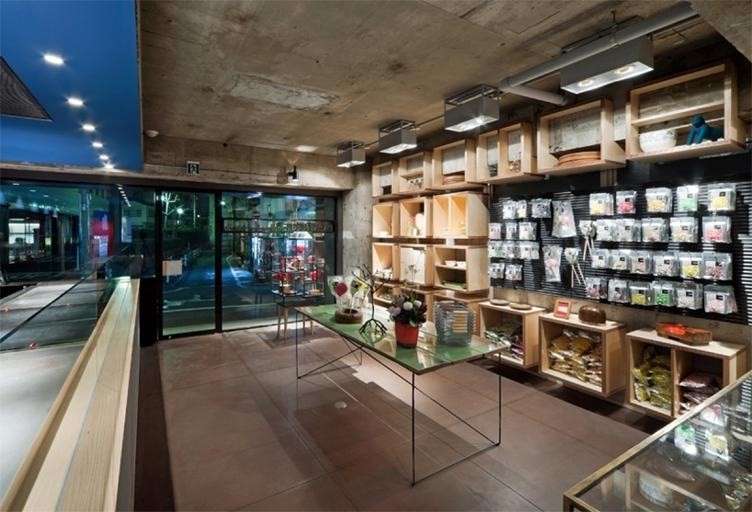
287;294;510;488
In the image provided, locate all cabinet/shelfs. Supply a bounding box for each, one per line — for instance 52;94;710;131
556;370;751;512
370;61;748;424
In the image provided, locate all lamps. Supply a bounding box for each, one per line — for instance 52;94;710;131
335;138;367;169
286;162;301;184
